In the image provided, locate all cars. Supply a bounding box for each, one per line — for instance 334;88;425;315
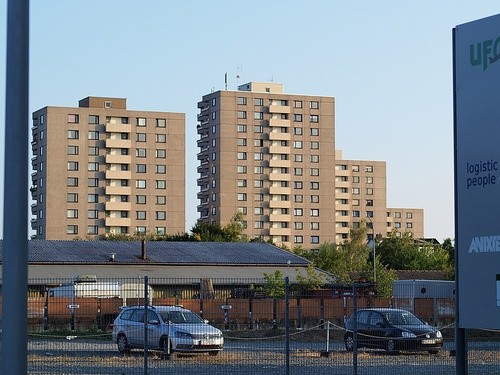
344;307;443;353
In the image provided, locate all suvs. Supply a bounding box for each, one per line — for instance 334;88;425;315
114;303;224;356
